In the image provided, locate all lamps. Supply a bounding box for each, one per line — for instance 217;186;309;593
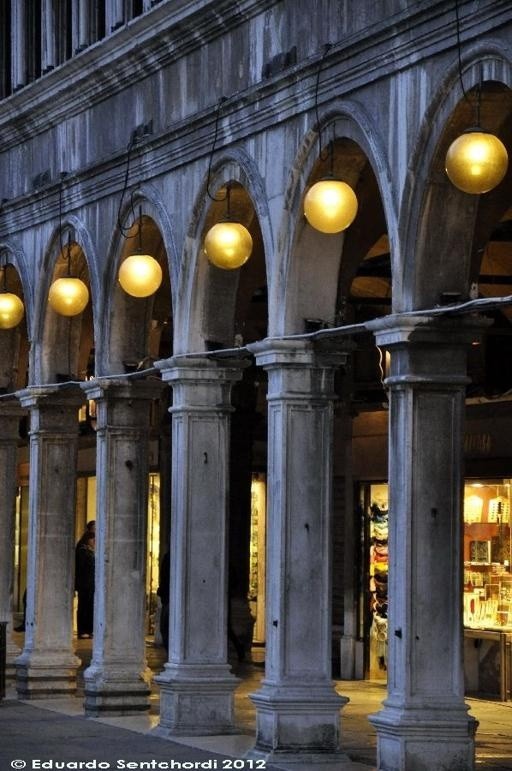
204;97;253;269
443;0;508;195
0;248;24;329
49;172;90;316
303;45;358;234
119;124;162;298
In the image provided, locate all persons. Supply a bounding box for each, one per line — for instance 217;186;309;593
76;532;95;639
158;550;170;607
77;520;96;545
229;628;245;659
13;588;26;632
